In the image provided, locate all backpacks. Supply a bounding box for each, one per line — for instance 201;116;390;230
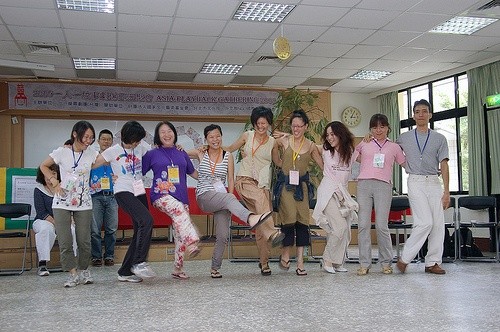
419;228;453;259
452;227;483;257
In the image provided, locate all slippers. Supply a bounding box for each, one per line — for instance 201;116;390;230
296;268;307;276
250;210;272;230
279;255;290;271
210;268;222;278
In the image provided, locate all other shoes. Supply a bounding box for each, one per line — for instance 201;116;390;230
425;263;445;274
320;260;336;274
188;248;200;258
357;267;369;275
396;260;406;274
271;232;285;248
37;266;49;276
170;272;189;279
259;262;271;276
333;264;348;272
379;266;392;274
91;258;102;267
104;258;114;266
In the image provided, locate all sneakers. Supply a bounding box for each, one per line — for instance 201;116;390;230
80;269;94;285
64;274;80;287
118;273;143;283
131;261;155;278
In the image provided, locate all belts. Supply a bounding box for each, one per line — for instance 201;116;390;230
100;190;113;196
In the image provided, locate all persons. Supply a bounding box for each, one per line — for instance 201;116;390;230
90;121;184;282
354;114;442;276
32;163;77;277
88;130;118;265
187;123;271;278
364;99;451;274
272;120;363;274
40;121;111;286
198;107;283;275
241;110;325;276
111;121;200;280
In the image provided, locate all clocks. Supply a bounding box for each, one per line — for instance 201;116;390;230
342;106;361;127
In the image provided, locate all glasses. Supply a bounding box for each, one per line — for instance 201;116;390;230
291;124;304;127
83;136;94;141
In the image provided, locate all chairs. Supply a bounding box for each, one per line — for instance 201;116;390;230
0;186;500;276
